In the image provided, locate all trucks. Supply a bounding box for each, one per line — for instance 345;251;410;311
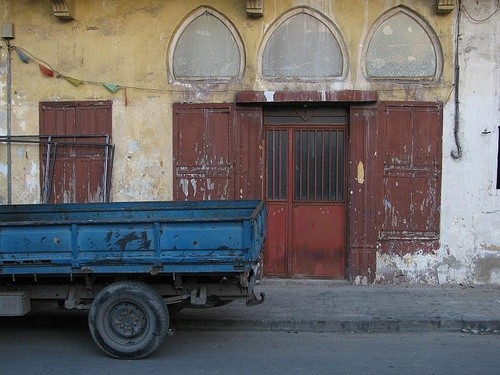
1;199;265;360
